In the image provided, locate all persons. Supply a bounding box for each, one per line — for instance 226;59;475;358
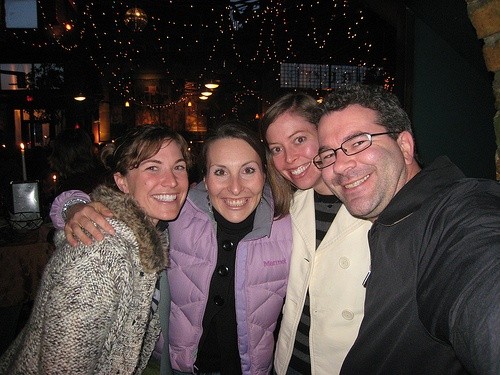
0;124;191;375
49;126;292;375
313;84;500;375
261;93;373;375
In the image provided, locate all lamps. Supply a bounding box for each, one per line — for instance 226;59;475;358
197;74;219;100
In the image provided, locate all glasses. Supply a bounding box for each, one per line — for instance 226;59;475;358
313;131;404;170
